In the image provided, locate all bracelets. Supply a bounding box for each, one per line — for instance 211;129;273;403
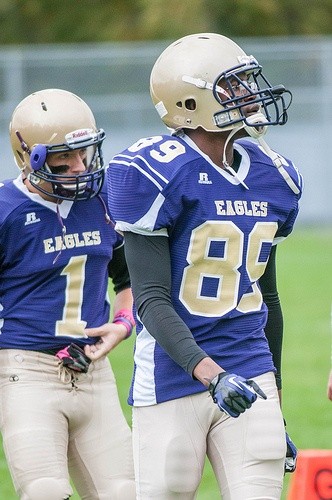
111;308;136;339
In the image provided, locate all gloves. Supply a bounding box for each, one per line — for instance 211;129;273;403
285;432;297;473
208;372;267;418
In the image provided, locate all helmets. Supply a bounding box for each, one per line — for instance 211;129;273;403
150;32;293;133
9;88;107;201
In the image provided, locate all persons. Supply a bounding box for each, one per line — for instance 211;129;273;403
105;31;305;500
0;88;135;500
